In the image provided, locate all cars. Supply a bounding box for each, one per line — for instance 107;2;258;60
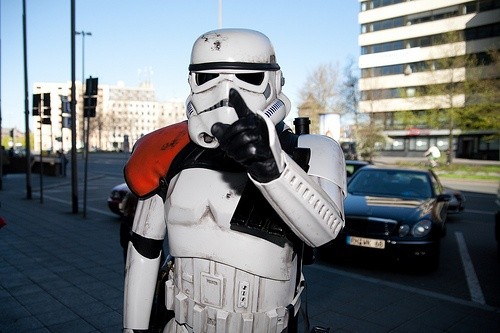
107;182;135;216
345;160;466;212
338;165;453;272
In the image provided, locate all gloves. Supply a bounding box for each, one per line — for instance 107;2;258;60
211;89;286;182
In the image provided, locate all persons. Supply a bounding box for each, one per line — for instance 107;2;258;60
0;146;67;177
401;179;427;198
423;147;440;168
445;149;450;165
122;27;347;333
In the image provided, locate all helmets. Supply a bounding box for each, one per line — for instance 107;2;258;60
187;30;291;148
